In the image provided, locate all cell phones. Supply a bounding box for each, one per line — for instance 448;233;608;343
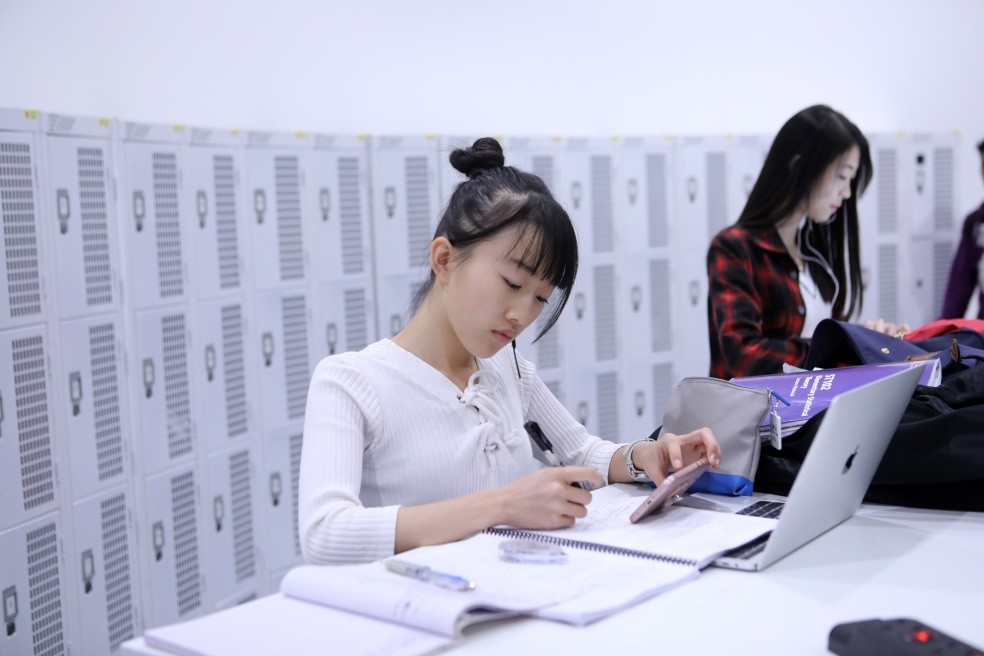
630;455;710;524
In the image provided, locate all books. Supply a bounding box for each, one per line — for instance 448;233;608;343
142;482;780;656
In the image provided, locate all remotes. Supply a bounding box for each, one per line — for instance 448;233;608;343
829;618;984;656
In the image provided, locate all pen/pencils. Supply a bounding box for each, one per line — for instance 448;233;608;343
386;559;476;591
524;421;581;488
499;553;568;563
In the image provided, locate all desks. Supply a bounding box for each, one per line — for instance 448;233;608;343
118;482;984;655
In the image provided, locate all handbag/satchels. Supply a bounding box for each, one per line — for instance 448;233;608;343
659;376;792;497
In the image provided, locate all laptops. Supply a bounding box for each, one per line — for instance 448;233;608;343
674;364;925;571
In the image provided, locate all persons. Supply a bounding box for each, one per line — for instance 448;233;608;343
298;137;721;564
708;104;907;381
941;138;984;320
623;436;654;486
